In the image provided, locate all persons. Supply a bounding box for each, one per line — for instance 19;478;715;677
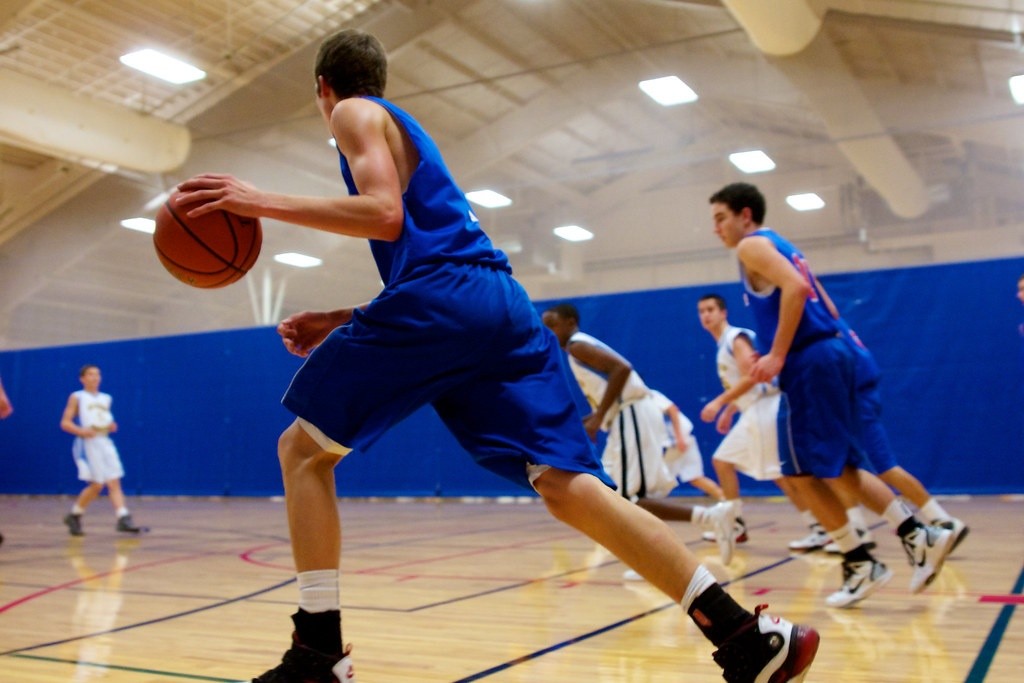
648;388;724;501
171;28;821;683
542;303;740;579
1017;276;1024;303
705;181;953;607
62;534;144;683
60;365;150;536
0;384;13;544
697;294;832;550
618;550;974;683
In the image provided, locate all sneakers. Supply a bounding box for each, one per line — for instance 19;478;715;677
903;525;954;592
714;499;738;564
931;515;968;550
64;513;83;536
825;528;876;553
242;631;354;683
789;527;829;550
712;603;820;683
702;519;747;542
116;515;139;533
827;554;892;606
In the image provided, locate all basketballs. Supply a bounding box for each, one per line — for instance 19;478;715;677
151;188;264;291
